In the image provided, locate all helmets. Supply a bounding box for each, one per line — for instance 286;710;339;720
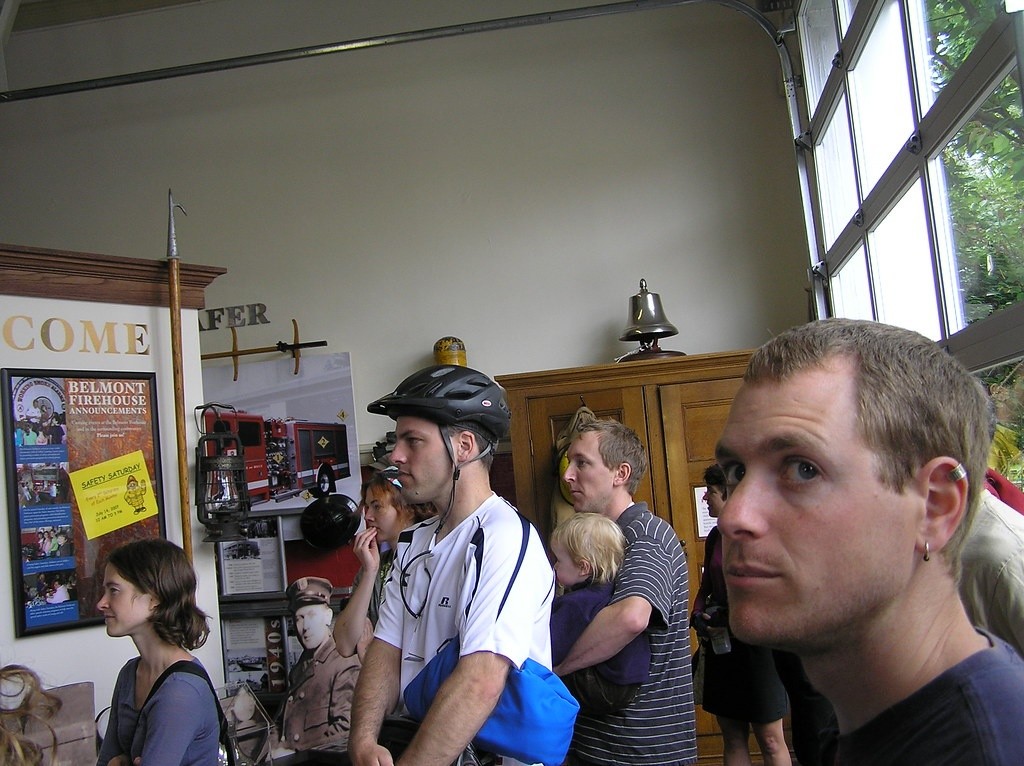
366;364;512;434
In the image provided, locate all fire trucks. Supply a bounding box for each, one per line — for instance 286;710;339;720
202;410;353;511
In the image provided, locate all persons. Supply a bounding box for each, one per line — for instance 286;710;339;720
714;318;1021;766
550;512;651;711
14;412;77;609
348;364;551;766
688;457;795;766
94;538;222;765
334;467;436;666
280;575;364;755
564;418;701;763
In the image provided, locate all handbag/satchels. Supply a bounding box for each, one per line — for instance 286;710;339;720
691;631;706;705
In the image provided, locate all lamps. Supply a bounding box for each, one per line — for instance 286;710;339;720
193;402;251;543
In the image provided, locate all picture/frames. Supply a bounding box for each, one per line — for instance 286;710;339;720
0;367;167;639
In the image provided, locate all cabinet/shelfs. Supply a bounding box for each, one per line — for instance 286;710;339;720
494;350;792;738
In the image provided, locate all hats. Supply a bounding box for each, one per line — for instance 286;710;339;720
286;576;333;611
